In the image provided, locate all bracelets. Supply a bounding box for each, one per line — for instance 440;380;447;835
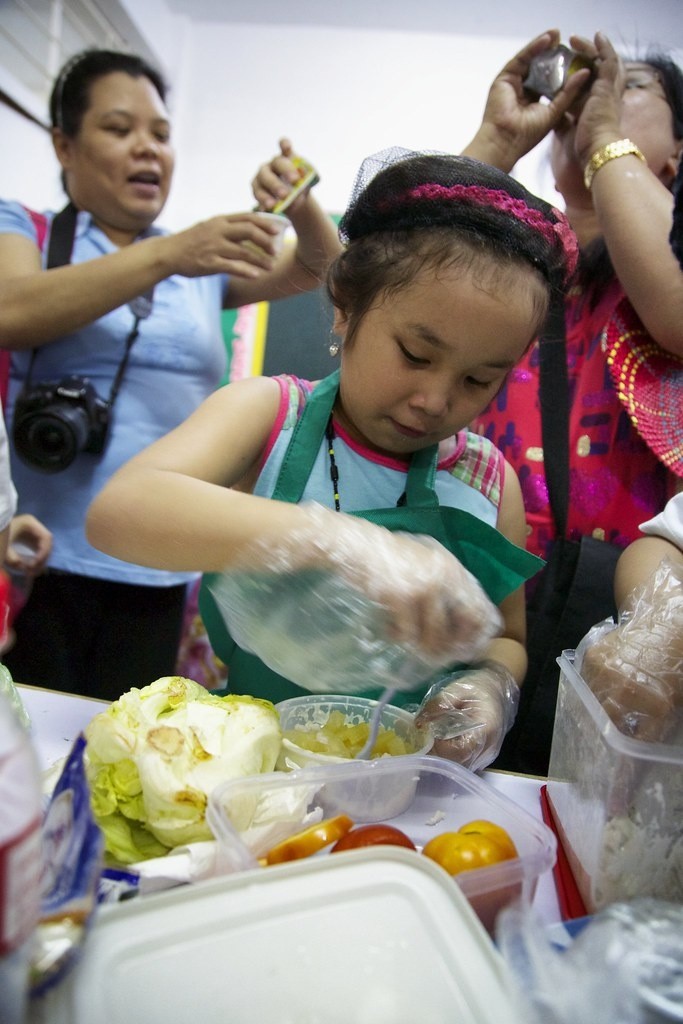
585;138;648;190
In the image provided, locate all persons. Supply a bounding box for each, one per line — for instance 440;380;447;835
581;492;681;816
87;155;576;775
453;30;683;779
0;50;347;702
0;391;54;657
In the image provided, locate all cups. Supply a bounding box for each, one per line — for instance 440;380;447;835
231;212;297;289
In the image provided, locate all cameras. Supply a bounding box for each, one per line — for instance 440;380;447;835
12;372;112;475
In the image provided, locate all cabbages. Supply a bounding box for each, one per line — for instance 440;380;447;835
83;676;280;864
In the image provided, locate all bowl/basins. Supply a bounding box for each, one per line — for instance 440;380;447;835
273;695;435;823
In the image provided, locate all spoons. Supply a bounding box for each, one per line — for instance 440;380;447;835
352;661;419;760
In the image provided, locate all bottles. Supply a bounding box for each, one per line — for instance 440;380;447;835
0;572;44;1024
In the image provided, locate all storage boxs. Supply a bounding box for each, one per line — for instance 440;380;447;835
27;844;514;1024
206;755;557;939
539;648;683;921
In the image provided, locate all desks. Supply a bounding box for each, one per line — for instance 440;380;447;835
14;682;560;921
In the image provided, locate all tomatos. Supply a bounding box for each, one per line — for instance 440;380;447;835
265;814;354;863
421;819;522;922
331;824;417;854
